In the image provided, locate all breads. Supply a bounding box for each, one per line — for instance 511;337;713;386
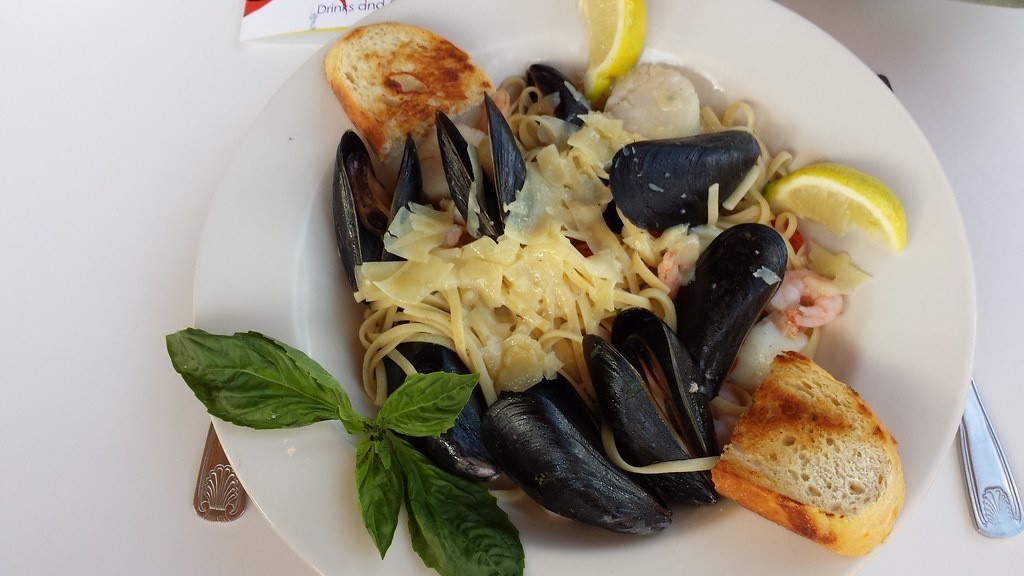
714;353;904;555
324;24;494;164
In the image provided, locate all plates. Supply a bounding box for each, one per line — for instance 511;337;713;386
193;0;977;575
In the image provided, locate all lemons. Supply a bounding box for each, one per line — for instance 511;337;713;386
766;162;907;257
577;1;646;101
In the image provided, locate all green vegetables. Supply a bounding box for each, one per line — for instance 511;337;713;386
165;328;527;576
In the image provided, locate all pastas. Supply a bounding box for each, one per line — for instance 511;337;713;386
357;75;866;474
